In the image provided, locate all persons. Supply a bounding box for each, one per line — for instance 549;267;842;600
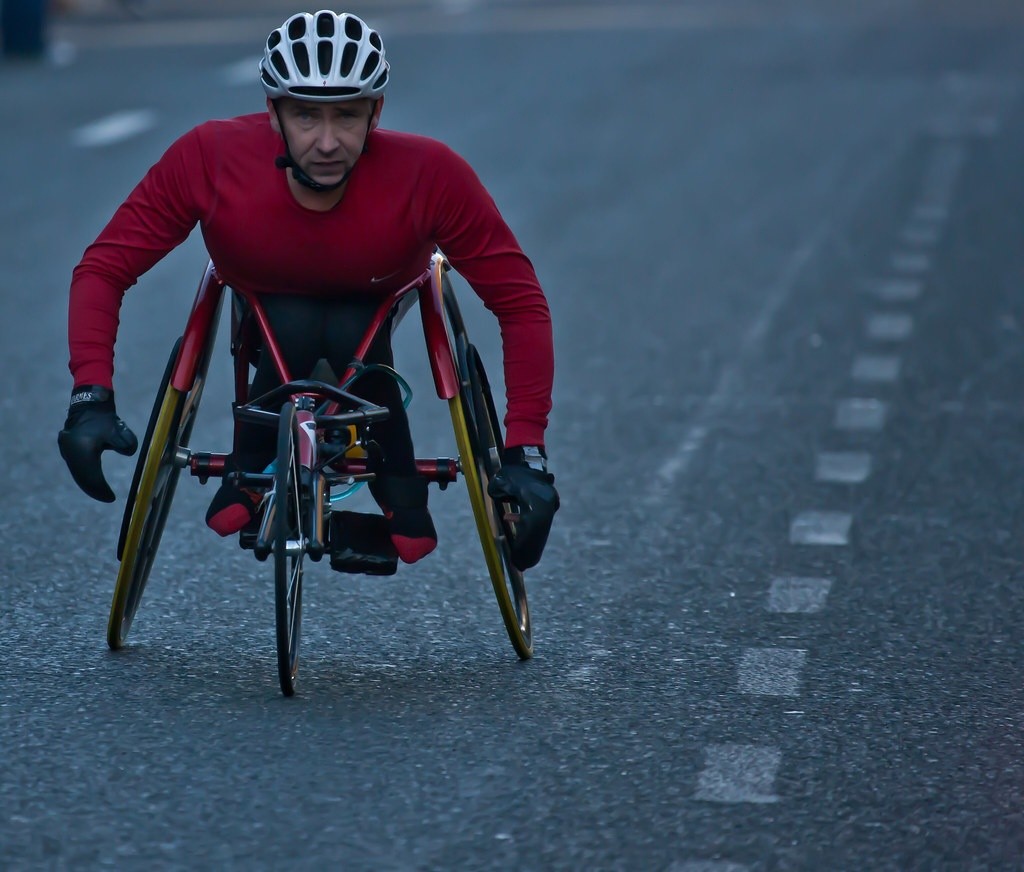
57;10;560;573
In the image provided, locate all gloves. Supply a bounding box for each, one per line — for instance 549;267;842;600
57;383;138;503
487;444;560;572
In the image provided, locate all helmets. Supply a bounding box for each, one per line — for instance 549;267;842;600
258;10;390;102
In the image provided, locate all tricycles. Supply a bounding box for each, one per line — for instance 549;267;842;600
107;252;534;695
